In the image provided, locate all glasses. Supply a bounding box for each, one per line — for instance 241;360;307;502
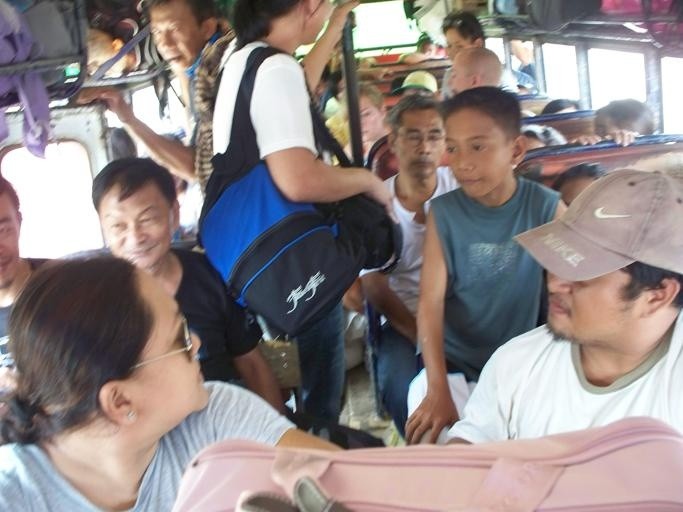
130;317;193;371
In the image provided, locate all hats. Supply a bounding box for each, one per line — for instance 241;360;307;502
388;70;438;96
513;168;683;283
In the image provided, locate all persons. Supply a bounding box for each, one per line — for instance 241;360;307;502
0;1;682;511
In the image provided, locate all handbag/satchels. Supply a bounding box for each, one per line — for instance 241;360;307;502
0;0;171;105
198;133;368;338
333;192;403;275
166;416;683;511
494;1;683;48
